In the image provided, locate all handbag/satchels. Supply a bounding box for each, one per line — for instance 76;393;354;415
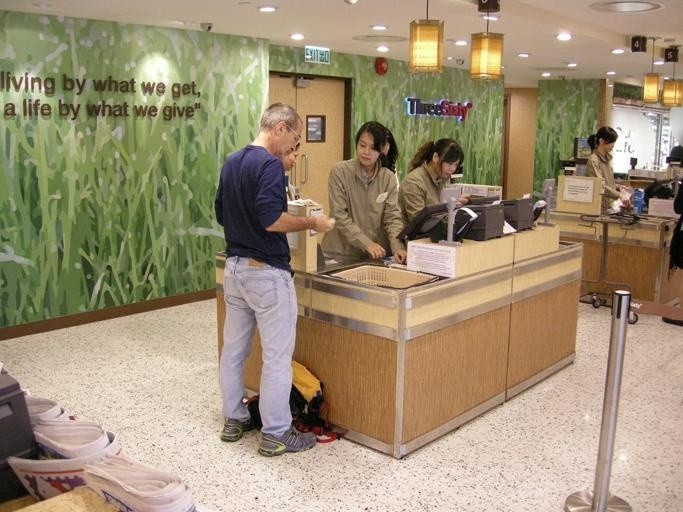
242;359;332;435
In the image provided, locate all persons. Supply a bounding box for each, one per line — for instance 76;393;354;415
321;121;413;264
584;126;632;210
214;103;335;457
398;138;471;235
281;143;301;171
669;177;683;274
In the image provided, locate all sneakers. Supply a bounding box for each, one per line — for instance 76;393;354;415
258;425;317;457
221;418;256;442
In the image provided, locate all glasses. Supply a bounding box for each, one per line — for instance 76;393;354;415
286;124;301;142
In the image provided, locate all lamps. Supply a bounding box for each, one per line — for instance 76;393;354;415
410;0;444;72
662;45;683;107
469;0;503;80
643;36;662;103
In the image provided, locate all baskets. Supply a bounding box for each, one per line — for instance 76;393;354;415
328;264;439;289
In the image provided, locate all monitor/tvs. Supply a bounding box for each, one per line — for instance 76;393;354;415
396;203;461;241
645;179;672;198
